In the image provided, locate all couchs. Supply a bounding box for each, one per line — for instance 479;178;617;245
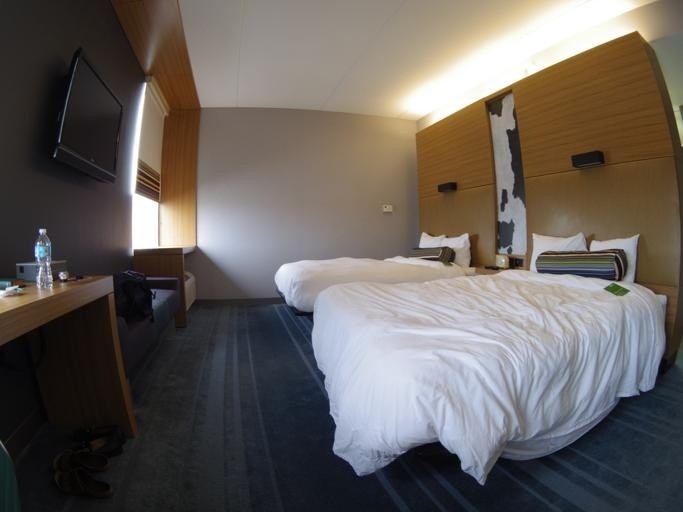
116;278;181;379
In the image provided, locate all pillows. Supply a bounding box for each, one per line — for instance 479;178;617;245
530;232;640;282
409;232;472;266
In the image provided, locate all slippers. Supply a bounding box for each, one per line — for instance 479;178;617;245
54;425;125;499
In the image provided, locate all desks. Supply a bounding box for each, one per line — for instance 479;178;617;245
134;247;197;329
0;274;138;439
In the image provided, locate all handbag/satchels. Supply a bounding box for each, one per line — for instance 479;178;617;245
117;270;152;319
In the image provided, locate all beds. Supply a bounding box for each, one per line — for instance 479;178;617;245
309;269;669;487
274;255;482;317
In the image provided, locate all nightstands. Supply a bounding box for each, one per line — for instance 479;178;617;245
477;255;526;275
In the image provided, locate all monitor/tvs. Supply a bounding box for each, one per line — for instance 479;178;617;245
47;46;124;184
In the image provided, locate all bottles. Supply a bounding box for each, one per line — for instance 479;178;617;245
32;229;54;290
